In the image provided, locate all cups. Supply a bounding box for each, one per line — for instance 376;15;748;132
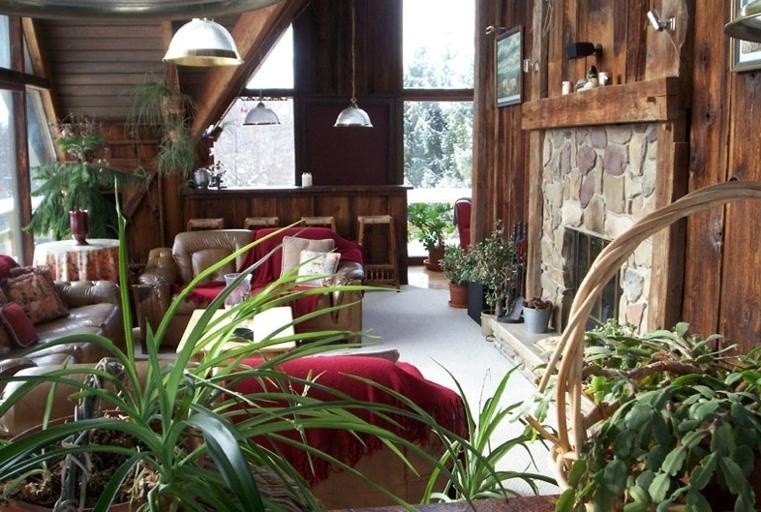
302;172;313;189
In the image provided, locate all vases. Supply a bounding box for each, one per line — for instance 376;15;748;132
69;209;89;244
521;300;552;333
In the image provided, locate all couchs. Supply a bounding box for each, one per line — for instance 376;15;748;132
0;255;126;394
132;227;366;354
1;356;477;508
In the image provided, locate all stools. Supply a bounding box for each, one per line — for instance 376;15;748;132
183;214;400;293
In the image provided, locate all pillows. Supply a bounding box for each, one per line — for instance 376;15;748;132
0;264;70;353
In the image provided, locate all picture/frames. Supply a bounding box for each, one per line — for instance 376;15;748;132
493;24;524;108
729;0;761;74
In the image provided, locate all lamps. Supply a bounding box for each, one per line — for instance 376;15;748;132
565;42;603;60
485;25;508;35
647;11;675;33
161;0;375;128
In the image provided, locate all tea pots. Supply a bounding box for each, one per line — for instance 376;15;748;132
223;273;252;309
194;167;210;189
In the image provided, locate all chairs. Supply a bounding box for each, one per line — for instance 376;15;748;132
453;198;471;254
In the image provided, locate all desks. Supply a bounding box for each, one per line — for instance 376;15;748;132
32;239;120;285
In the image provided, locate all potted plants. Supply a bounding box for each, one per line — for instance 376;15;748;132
407;203;453;264
439;219;526;337
524;181;761;512
0;174;510;512
